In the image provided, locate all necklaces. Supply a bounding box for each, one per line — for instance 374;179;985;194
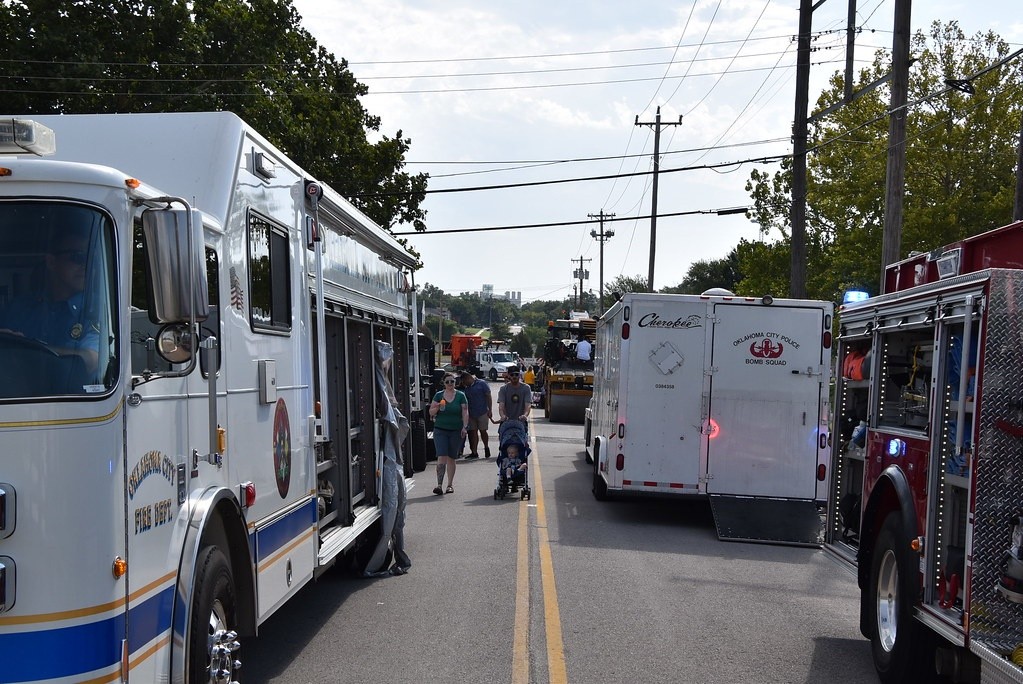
509;458;516;465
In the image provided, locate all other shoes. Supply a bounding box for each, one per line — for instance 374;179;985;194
508;477;513;484
433;487;443;495
465;452;479;459
484;447;490;458
511;486;518;492
446;486;454;493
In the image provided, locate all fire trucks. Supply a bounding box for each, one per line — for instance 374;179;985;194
814;219;1023;684
583;287;835;548
0;107;423;684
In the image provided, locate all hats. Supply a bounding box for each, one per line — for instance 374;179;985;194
508;366;520;374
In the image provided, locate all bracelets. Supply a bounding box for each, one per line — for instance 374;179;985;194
464;424;469;429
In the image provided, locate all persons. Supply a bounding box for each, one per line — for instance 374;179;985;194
523;366;537;390
0;207;100;376
429;372;469;495
489;366;532;493
574;337;592;362
502;444;527;484
460;371;492;459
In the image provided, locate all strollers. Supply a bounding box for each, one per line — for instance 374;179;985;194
493;417;534;501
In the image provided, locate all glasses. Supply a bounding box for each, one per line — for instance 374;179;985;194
510;373;520;377
444;380;455;384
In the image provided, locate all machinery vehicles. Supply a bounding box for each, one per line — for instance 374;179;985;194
448;333;522;382
538;308;599;425
406;333;445;471
